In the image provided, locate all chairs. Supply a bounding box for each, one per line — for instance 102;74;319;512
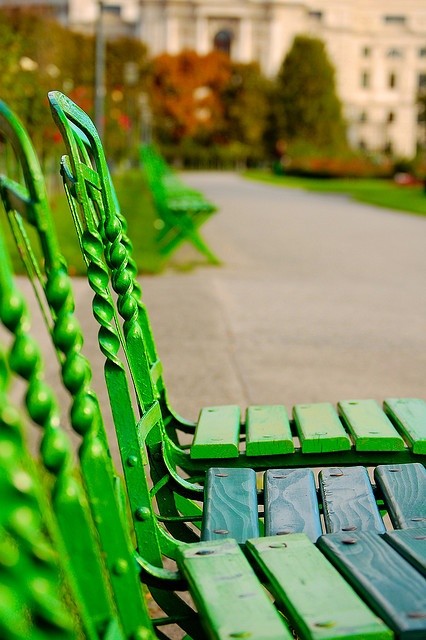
0;99;426;640
136;142;220;265
59;154;419;533
47;91;424;537
